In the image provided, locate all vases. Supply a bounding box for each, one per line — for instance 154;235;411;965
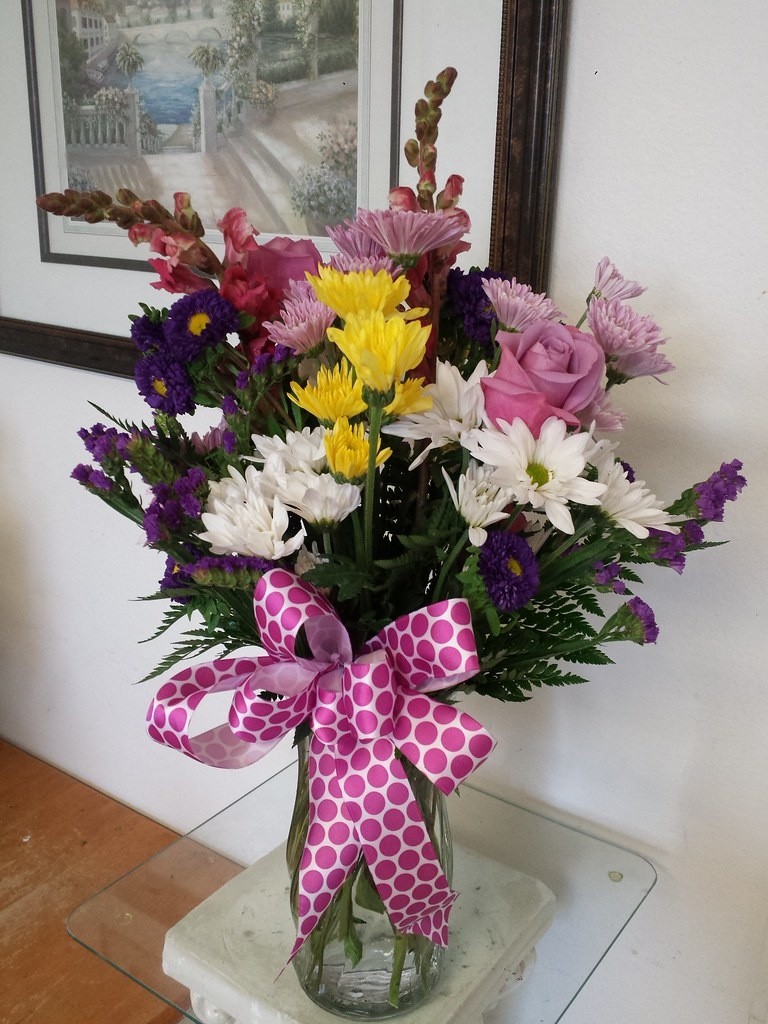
272;677;458;1017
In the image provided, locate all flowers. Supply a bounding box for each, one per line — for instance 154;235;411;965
39;64;748;707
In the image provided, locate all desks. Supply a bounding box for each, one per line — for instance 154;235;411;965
62;750;659;1023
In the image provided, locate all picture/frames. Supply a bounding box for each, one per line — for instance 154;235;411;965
21;0;404;278
